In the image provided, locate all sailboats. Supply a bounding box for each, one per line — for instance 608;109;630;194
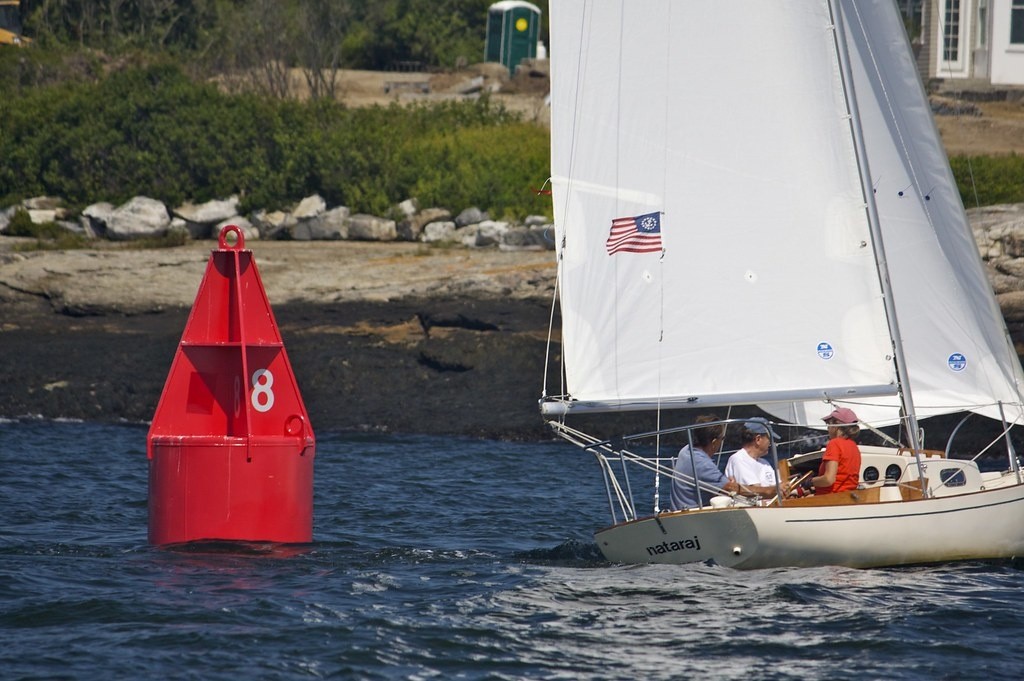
538;0;1024;571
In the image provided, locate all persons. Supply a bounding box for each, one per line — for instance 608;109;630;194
668;412;791;511
724;417;782;487
798;408;861;496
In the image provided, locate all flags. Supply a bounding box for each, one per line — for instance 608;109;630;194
606;211;663;256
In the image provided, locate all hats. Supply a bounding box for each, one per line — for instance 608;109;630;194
820;408;859;424
743;417;781;439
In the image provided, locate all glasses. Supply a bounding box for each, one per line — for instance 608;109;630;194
718;436;726;441
